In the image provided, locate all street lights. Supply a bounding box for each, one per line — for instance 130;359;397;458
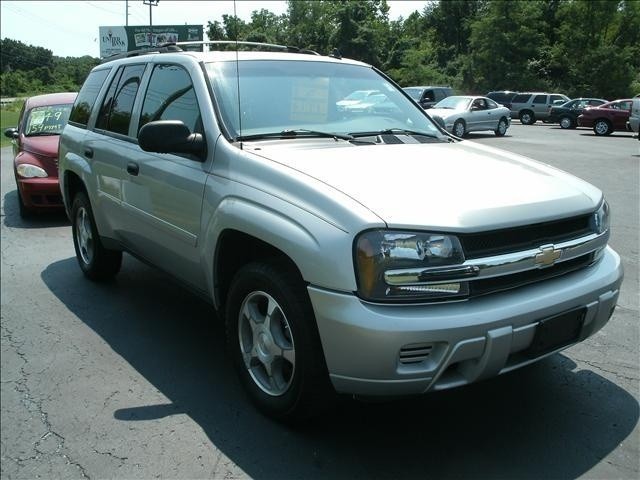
142;0;159;48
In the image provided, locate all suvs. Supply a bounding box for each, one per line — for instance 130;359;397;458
335;85;455;118
58;39;623;410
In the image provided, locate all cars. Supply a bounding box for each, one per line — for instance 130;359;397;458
423;96;511;137
486;91;640;138
4;92;79;220
